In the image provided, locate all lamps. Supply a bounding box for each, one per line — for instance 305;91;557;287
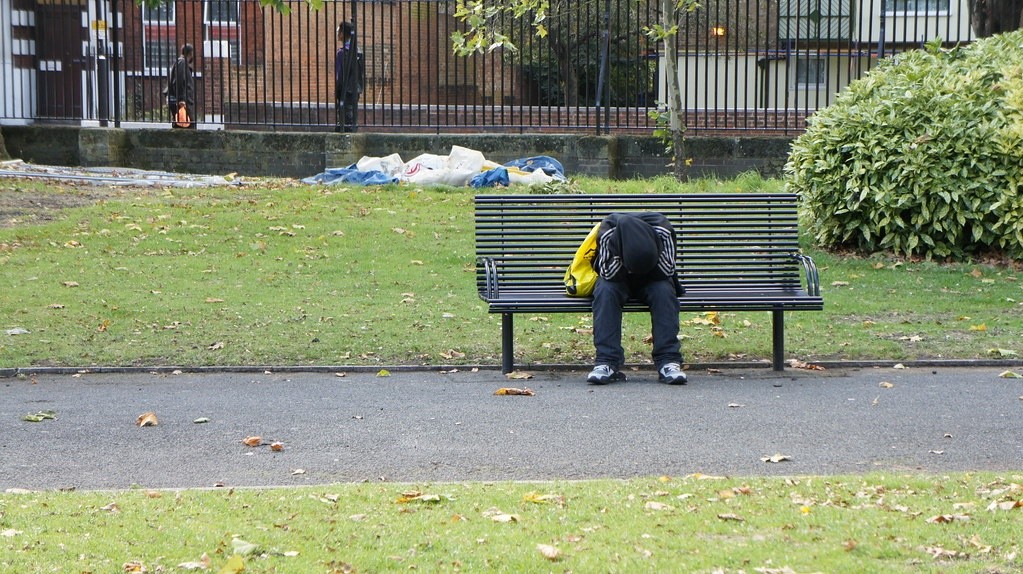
713;23;724;37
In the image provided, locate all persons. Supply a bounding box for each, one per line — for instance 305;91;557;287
163;44;195;129
587;211;687;385
335;21;364;133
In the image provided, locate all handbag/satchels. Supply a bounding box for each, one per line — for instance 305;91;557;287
175;107;191;127
564;222;601;297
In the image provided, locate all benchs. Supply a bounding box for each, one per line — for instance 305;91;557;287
473;194;824;371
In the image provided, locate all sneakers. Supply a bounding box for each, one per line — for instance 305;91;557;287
659;362;687;384
587;364;620;384
